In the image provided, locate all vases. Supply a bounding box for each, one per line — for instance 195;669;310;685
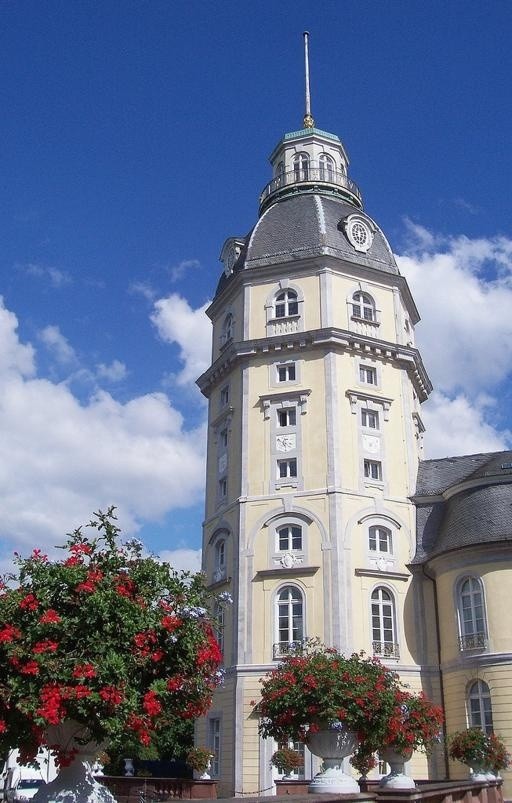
25;711;122;803
281;769;293;780
469;767;501;780
123;758;136;777
378;743;416;790
190;769;213;781
357;771;370;780
302;723;361;793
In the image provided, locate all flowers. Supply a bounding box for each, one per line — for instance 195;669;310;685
247;631;444;757
0;503;234;772
271;748;306;771
186;744;210;770
351;752;380;771
450;726;511;771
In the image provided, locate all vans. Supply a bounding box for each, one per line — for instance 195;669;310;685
6;764;48;802
